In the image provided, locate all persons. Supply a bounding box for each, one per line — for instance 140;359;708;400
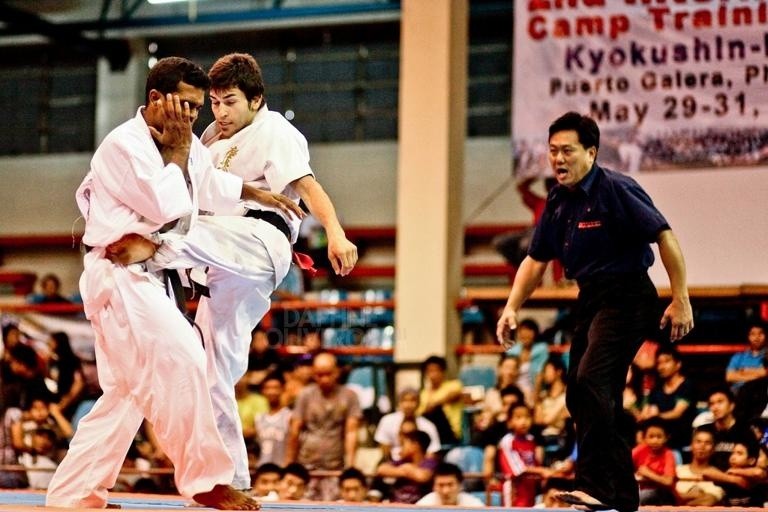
492;170;576;288
42;53;308;512
1;275;768;512
104;54;357;490
493;112;694;509
511;123;768;171
